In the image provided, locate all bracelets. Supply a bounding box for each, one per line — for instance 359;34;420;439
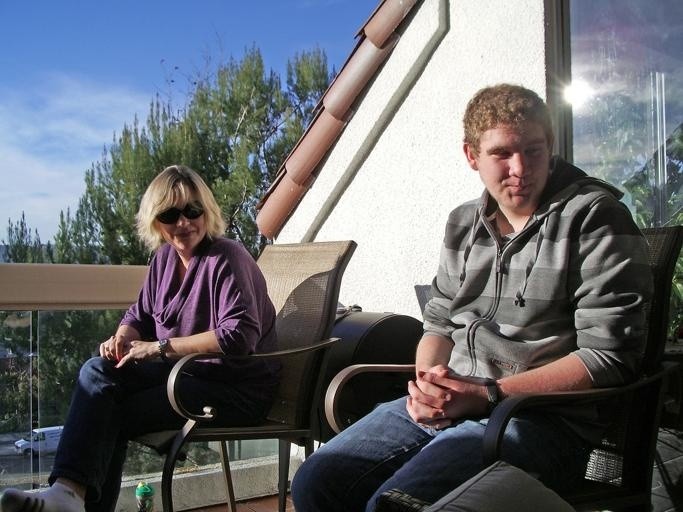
485;379;498;417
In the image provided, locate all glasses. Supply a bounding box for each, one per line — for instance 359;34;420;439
155;200;205;225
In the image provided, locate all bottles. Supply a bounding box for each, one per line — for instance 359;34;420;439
134;482;154;512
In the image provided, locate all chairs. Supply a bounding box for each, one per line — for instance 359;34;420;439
125;239;359;512
322;223;683;512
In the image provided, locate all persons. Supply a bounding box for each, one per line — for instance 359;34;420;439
0;165;283;512
290;84;655;512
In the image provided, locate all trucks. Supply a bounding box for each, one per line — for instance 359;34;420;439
15;423;67;459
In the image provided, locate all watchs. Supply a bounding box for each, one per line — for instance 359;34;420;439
159;338;168;360
